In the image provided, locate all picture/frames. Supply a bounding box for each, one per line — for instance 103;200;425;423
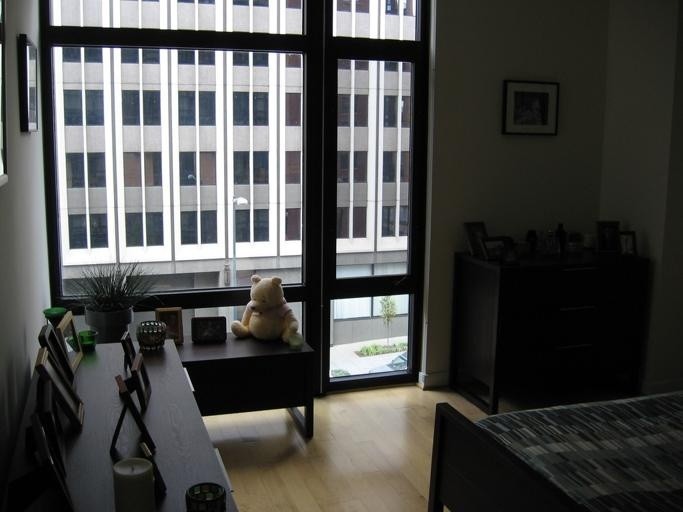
53;311;86;377
109;375;156;452
119;306;227;414
28;376;70;476
14;31;39;136
7;414;75;512
500;77;559;139
34;346;86;434
459;220;637;265
1;1;10;186
38;324;74;385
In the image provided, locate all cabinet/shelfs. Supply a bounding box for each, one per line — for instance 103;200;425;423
447;250;651;417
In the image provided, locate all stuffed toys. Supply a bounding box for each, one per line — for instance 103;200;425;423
231;274;304;349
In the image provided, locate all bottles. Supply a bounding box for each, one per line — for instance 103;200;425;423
525;222;568;256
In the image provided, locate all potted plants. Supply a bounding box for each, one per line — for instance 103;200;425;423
67;266;164;342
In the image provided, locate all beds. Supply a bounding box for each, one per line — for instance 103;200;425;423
427;390;681;511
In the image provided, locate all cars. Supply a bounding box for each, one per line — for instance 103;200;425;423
368;350;408;373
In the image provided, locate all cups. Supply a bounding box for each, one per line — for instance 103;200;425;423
135;321;166;350
184;481;226;512
43;308;65;326
77;330;97;352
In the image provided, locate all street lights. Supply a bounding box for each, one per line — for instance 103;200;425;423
228;196;249;325
187;173;197;184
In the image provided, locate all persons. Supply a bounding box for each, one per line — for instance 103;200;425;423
165;313;178;338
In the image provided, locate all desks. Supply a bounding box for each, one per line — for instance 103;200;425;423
2;337;238;512
175;332;316;438
